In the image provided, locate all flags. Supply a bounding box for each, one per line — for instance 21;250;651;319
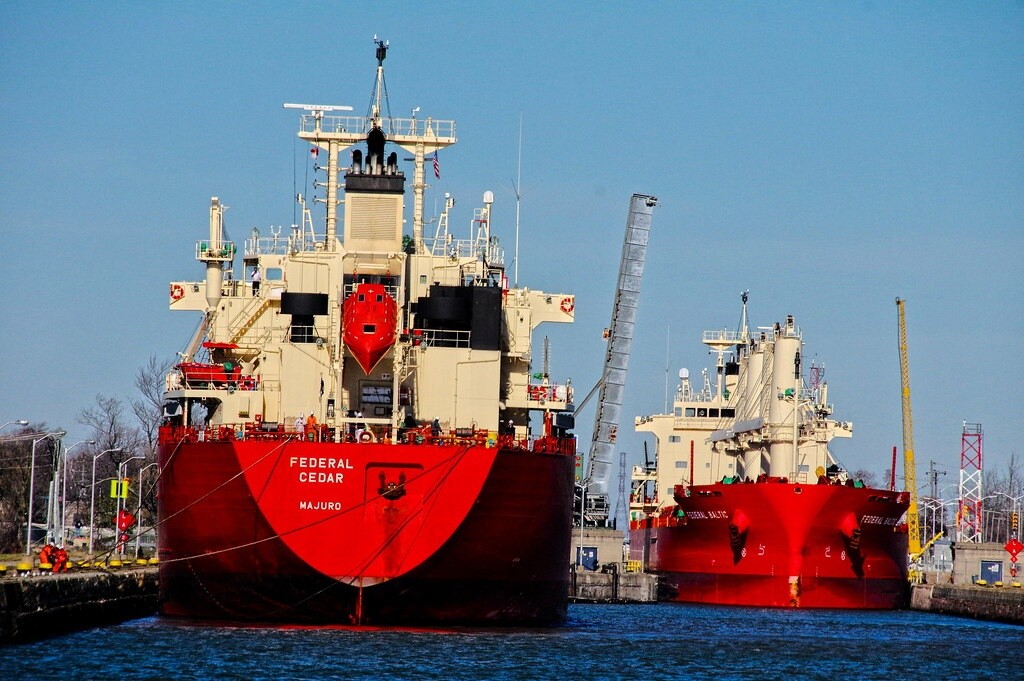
433;150;440;179
311;146;319;158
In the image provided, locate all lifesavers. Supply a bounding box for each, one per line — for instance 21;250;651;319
170;284;184;299
560;298;574;313
239;376;255;391
359;431;373;443
346;434;354;443
532;386;547;401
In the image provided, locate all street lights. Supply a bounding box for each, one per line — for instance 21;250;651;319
114;455;146;554
26;432;65;554
136;463;158;556
916;484;996;546
89;447;124;554
993;491;1017;581
61;439;95;546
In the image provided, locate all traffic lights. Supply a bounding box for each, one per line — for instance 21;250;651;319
1012;512;1018;531
964;505;968;517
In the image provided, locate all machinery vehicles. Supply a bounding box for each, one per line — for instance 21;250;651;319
574;193;657;526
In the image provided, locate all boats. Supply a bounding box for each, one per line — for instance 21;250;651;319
153;36;580;632
624;289;914;609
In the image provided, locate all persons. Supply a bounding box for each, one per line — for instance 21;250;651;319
39;542;69;573
346;406;365;439
499;420;515;440
404;411;417;427
296;411;317;431
431;417;442;436
251;267;261;297
